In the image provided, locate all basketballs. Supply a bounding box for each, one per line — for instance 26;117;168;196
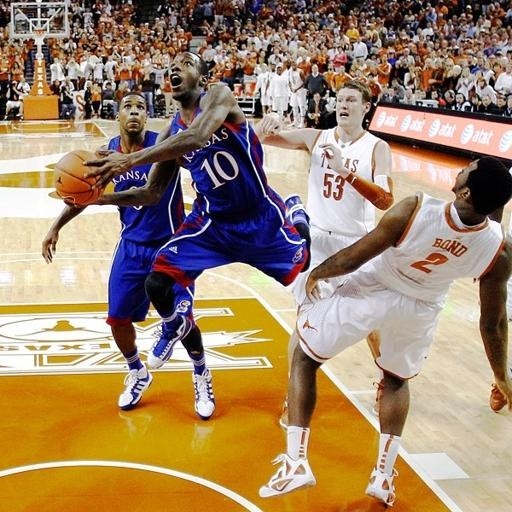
54;150;106;206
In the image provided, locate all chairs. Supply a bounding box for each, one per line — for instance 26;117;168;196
0;1;511;122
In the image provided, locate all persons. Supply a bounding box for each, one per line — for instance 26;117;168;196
60;48;312;372
39;92;218;420
254;157;511;508
252;77;396;429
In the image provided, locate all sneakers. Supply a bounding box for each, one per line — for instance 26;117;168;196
257;454;321;499
143;315;196;372
363;465;398;508
116;368;154;411
188;368;219;419
